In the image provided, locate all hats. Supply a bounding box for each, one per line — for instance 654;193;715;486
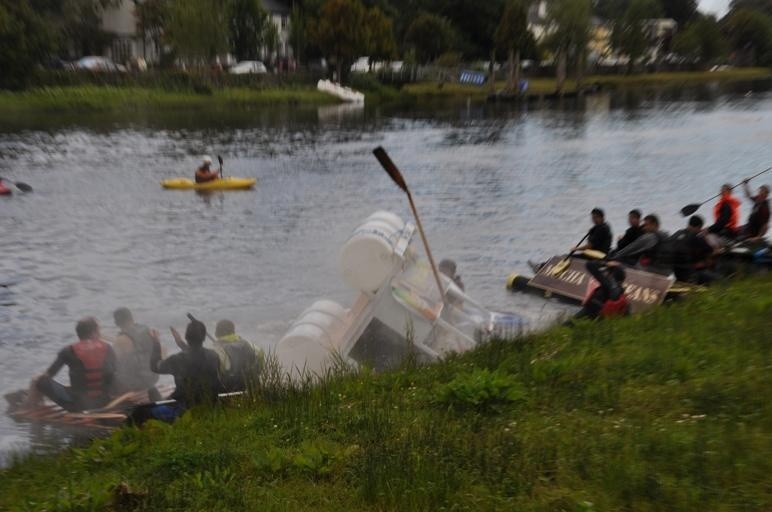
203;157;212;165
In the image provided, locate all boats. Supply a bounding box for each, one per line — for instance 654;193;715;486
450;303;533;338
4;381;255;429
159;176;258;191
668;278;707;299
701;227;771;264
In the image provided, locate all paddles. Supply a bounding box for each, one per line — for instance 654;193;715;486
170;326;187;350
552;231;590;275
681;167;772;216
218;154;223;179
372;145;448;307
118;389;253;411
0;177;32;191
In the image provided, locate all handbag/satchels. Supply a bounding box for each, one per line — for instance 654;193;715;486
126;400;185;427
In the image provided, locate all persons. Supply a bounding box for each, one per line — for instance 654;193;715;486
25;315;116;413
439;259;465;293
124;321;220;427
195;155;220;181
113;306;159;394
210;320;264;388
565;177;772;325
